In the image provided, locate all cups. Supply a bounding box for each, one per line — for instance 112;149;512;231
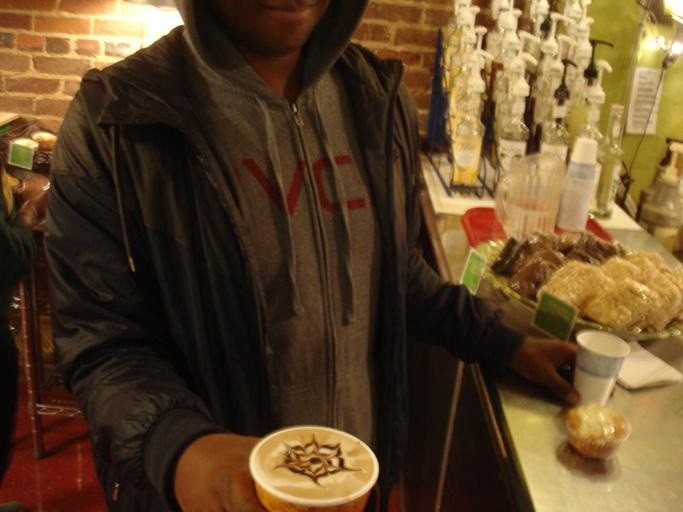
496;155;565;241
247;425;380;511
573;331;632;404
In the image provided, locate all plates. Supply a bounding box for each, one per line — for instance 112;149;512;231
472;238;683;342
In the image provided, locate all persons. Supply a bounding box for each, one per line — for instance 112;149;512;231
0;139;51;511
42;0;618;511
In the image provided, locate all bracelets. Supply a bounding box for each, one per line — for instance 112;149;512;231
21;200;41;227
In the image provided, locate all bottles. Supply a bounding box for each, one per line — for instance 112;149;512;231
555;105;623;232
